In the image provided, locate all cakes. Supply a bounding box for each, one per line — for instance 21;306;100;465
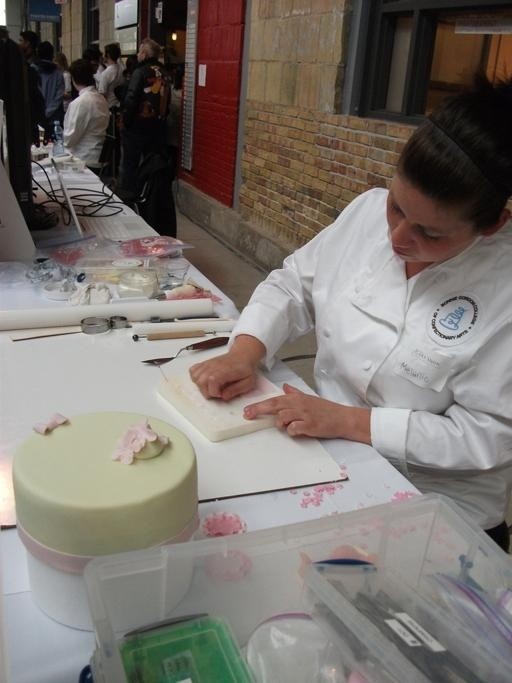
14;411;197;571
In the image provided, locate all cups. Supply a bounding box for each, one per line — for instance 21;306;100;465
156;258;190;290
63;157;81;176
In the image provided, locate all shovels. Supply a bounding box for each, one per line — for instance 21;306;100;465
142;337;230;365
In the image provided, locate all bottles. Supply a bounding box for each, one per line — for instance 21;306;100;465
52;121;64;156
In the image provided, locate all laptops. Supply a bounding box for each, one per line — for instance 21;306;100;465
49;158;160;243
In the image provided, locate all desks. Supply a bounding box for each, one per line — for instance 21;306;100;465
0;139;512;683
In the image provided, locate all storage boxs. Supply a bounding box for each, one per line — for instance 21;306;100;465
83;490;511;683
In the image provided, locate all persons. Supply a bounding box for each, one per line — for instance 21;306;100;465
189;65;512;553
1;24;183;239
